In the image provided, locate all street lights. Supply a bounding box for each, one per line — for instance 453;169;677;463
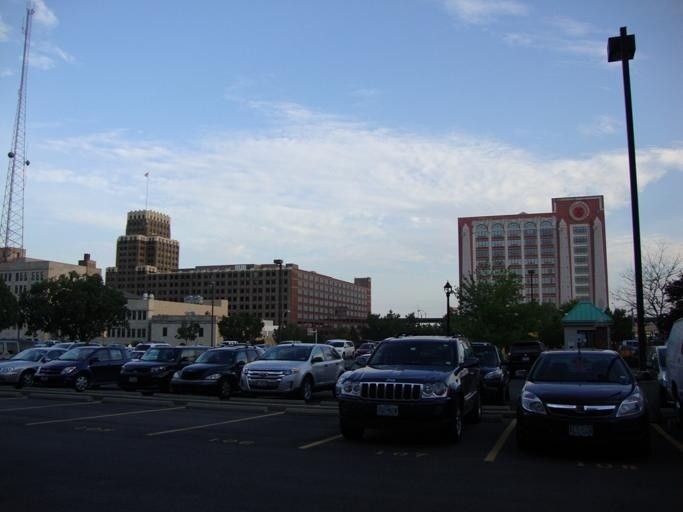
604;27;652;370
274;258;283;318
416;308;427;318
279;308;290;326
443;280;454;335
209;280;215;347
527;269;536;301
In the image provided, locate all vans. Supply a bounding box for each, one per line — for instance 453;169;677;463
664;317;682;408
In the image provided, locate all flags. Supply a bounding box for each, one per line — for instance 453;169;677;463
144;171;149;176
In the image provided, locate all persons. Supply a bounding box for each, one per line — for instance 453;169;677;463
46;338;55;347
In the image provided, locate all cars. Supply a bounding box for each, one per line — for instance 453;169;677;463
238;341;345;404
33;344;133;393
623;338;639;348
650;345;667;388
469;339;512;404
0;347;67;387
0;338;171;366
221;338;378;361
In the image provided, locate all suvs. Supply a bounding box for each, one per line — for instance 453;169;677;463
507;339;543;377
330;334;486;446
171;344;267;402
517;346;650;450
118;345;209;390
